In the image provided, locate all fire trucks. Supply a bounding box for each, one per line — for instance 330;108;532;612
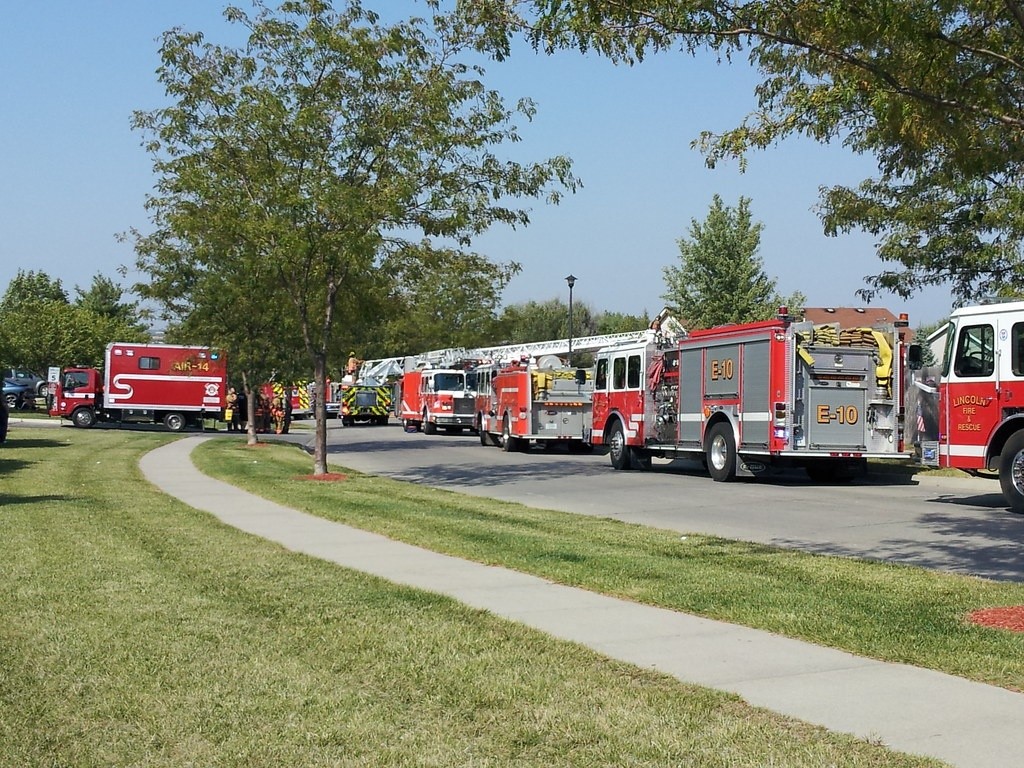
262;330;661;435
913;302;1024;514
475;354;594;455
590;306;926;483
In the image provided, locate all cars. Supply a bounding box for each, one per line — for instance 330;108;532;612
4;368;49;398
2;380;33;409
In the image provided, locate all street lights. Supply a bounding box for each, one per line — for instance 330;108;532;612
564;274;578;368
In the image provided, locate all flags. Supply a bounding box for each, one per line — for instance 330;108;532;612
916;399;925;432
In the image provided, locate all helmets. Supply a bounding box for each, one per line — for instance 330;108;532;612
349;351;355;357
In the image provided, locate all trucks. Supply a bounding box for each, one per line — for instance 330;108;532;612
49;342;233;433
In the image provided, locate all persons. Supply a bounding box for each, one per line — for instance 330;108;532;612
226;384;285;434
653;321;663;350
348;352;365;385
